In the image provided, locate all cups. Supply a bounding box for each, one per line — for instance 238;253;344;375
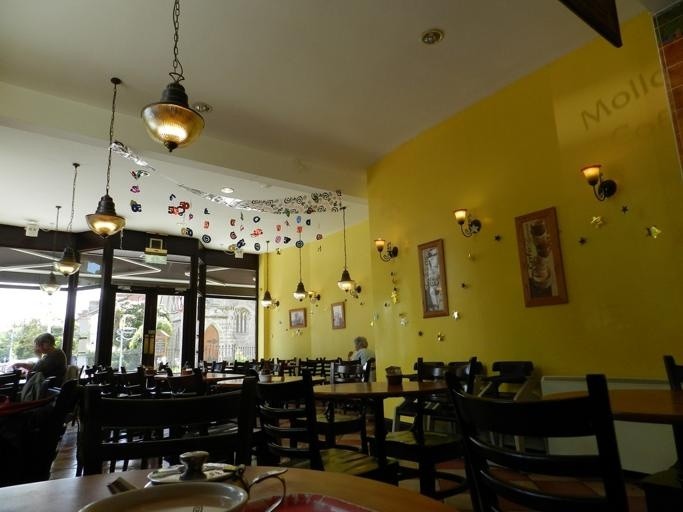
386;375;402;384
258;373;272;382
75;476;248;512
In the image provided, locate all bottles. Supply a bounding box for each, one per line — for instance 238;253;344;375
260;362;269;373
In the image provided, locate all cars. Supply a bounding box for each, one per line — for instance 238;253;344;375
0;360;27;379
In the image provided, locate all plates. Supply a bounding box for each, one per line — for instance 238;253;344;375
242;492;370;512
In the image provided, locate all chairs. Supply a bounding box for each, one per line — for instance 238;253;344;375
0;355;683;512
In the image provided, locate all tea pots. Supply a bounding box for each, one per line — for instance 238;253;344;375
106;451;289;512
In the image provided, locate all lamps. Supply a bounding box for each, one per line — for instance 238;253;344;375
336;205;357;295
260;237;272;313
36;205;64;298
578;160;619;202
451;205;482;238
51;162;81;279
83;75;128;242
291;226;309;304
138;1;206;159
373;236;398;262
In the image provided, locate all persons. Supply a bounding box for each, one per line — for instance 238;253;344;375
347;336;376;383
11;332;68;389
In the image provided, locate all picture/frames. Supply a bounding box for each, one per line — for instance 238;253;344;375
288;307;308;330
414;237;450;320
512;205;571;308
329;301;346;330
